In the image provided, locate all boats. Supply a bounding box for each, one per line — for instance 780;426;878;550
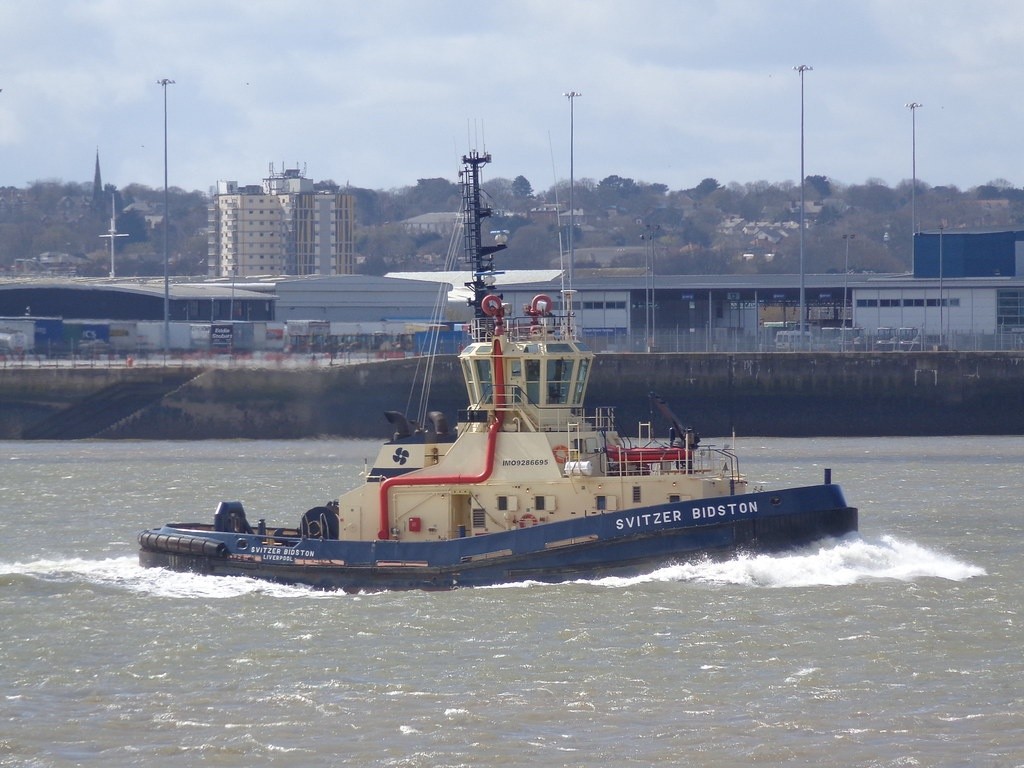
137;135;859;590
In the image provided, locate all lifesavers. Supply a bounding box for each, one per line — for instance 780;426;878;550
553;444;569;464
519;513;538;528
480;294;502;317
531;294;552;313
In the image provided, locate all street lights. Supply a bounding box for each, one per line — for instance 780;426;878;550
905;102;926;279
791;63;813;329
840;233;858;349
153;77;176;356
560;90;584;288
939;223;947;348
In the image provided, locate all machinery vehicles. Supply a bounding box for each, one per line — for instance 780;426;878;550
775;327;920;350
